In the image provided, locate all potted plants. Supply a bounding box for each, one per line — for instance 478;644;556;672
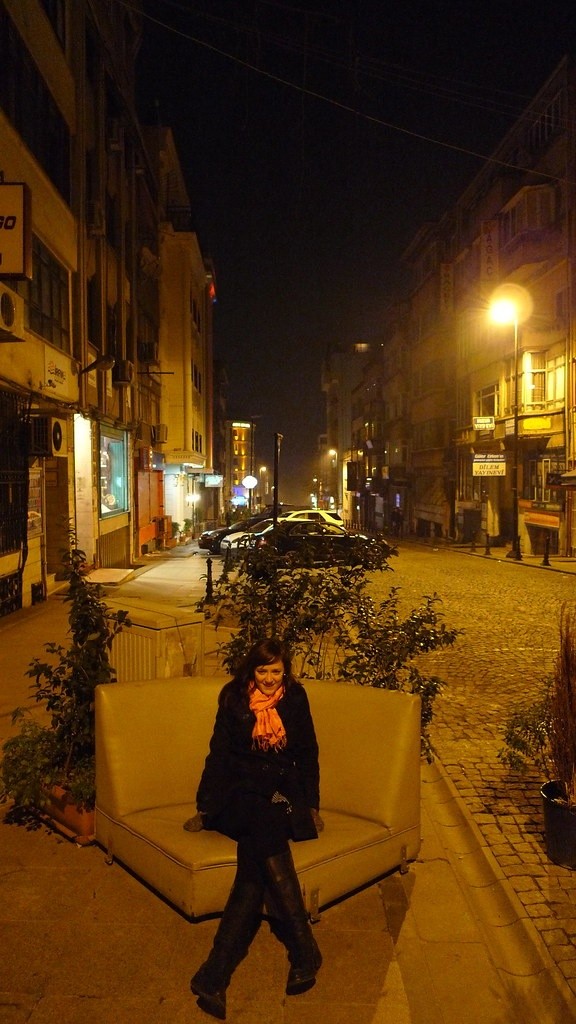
0;515;132;845
540;601;576;867
172;518;201;548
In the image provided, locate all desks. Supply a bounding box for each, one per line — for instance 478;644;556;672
82;568;134;598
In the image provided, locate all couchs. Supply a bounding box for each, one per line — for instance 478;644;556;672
93;681;422;923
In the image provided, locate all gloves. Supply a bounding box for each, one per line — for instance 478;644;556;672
183;811;207;831
308;806;325;832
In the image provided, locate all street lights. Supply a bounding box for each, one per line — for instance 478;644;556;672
259;467;267;515
329;447;339;513
488;296;523;559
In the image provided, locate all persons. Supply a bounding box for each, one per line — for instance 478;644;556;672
184;639;328;1015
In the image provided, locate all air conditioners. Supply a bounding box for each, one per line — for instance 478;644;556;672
32;417;68;458
147;342;159;364
155;424;167;442
119;360;134;384
0;283;24;343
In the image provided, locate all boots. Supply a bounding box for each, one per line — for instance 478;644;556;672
190;878;263;1017
260;850;322;986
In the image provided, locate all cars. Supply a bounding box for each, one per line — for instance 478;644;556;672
221;517;318;561
247;520;391;569
280;509;345;528
197;518;266;554
